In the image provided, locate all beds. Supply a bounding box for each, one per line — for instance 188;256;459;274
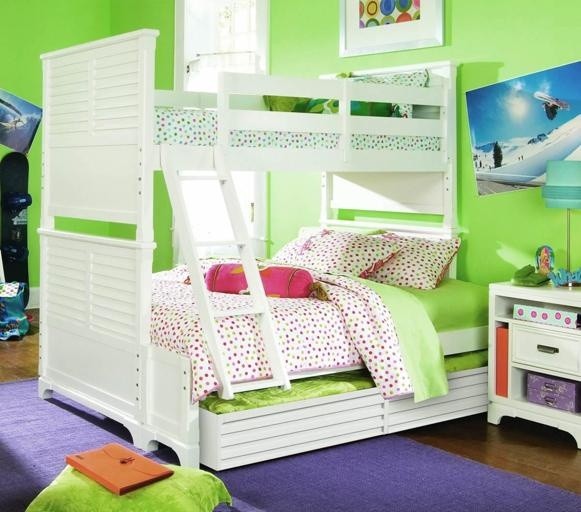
38;30;503;470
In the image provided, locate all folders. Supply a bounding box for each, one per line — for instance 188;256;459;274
67;442;174;496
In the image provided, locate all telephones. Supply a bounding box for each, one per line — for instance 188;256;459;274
511;265;551;287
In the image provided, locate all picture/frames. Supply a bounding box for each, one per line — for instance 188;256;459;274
339;0;443;57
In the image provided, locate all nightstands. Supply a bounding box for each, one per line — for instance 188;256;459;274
487;281;581;450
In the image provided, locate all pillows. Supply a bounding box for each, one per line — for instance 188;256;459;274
272;225;403;280
364;232;461;290
202;264;329;301
348;69;428;118
24;463;233;512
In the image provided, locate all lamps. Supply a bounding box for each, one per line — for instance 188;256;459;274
542;160;581;286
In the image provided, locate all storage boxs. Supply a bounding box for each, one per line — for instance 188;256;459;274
525;372;578;411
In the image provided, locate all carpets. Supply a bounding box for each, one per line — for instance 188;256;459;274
0;378;581;511
